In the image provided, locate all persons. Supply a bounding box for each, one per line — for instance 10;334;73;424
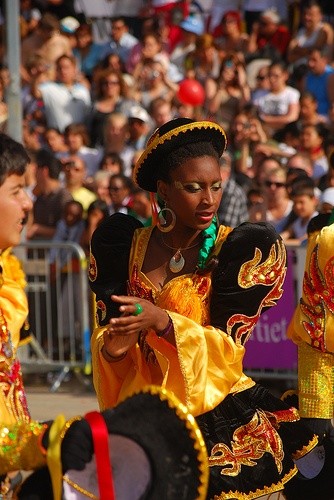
0;0;334;500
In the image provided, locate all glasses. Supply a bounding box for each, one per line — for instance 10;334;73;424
265;180;287;186
103;81;119;87
107;186;123;190
111;24;126;30
255;76;267;80
246;200;260;207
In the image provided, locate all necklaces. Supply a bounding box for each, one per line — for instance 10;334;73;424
158;229;201;274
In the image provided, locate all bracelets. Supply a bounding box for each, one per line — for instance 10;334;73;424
155;319;174;339
101;346;128;363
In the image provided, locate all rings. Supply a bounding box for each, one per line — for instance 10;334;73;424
132;304;144;314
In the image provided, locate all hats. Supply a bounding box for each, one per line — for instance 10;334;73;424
132;118;227;193
16;385;209;500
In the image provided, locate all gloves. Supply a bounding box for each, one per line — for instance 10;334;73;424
42;419;95;472
